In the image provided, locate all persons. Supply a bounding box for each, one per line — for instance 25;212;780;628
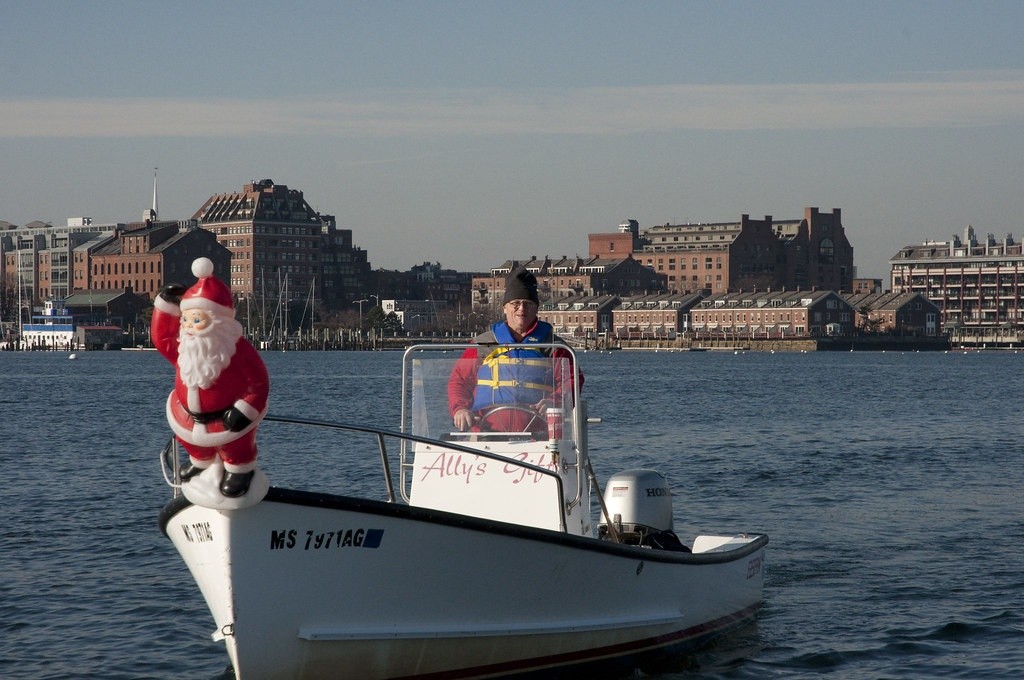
151;256;268;499
448;265;584;441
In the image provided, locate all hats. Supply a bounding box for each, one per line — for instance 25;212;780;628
503;265;540;308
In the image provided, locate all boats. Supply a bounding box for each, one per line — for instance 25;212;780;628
160;344;769;679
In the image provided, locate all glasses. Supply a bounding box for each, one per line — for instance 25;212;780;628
508;302;536;309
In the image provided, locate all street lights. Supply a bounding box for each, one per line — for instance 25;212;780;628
353;299;368;328
370;294;378;306
410;315;420;333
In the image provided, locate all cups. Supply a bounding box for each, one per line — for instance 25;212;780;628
546;407;564;443
